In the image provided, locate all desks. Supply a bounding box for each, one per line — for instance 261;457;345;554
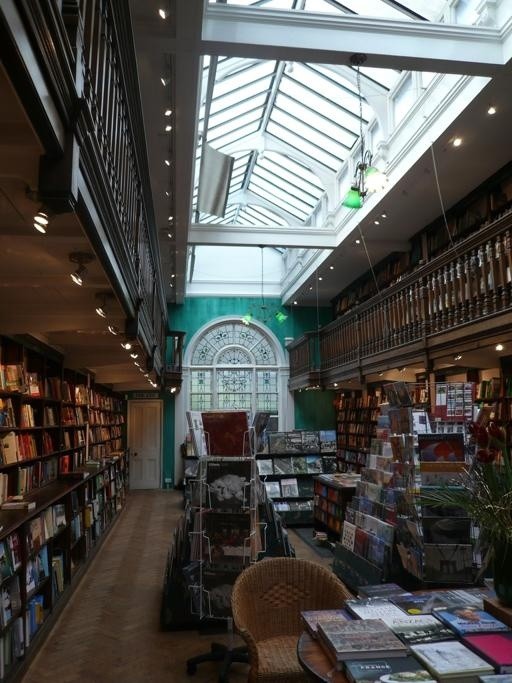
296;584;512;683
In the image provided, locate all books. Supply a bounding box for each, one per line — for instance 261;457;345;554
180;411;297;617
1;362;85;682
86;388;129;541
317;381;512;583
254;431;338;523
300;584;512;683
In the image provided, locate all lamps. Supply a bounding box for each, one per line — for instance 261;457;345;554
33;206;160;392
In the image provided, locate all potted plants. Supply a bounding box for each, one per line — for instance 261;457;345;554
394;430;512;609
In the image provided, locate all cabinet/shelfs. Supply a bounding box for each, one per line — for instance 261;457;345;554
1;330;131;681
256;349;511;589
159;437;298;638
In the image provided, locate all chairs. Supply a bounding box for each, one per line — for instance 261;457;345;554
229;556;359;682
